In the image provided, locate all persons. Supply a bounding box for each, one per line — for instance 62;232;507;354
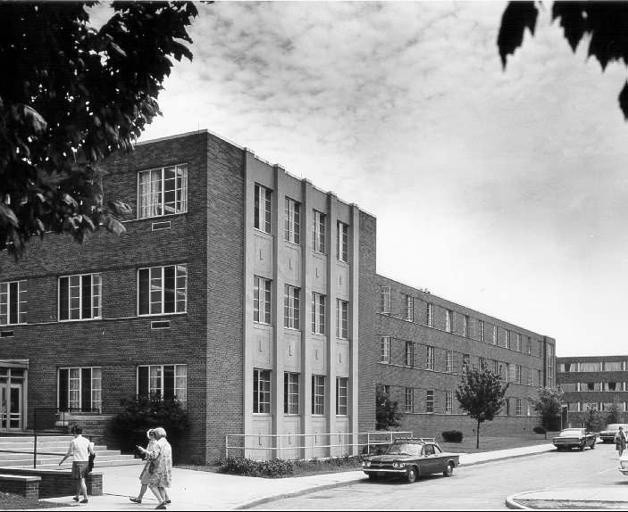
613;426;628;460
137;427;174;509
58;424;96;502
128;428;171;504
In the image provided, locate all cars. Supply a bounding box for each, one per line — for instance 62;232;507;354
361;439;461;482
552;422;628;452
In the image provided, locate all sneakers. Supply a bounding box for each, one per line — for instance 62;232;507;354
130;498;141;503
156;499;171;509
74;497;88;503
